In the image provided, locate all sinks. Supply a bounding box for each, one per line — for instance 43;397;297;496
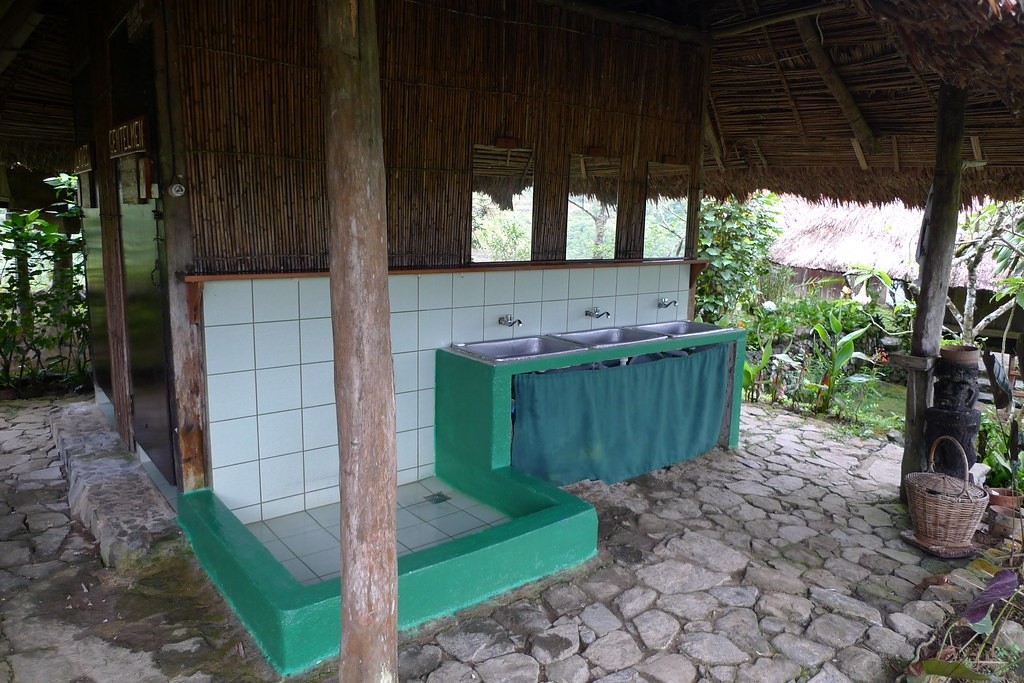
546;326;665;348
452;336;589;361
627;319;734;338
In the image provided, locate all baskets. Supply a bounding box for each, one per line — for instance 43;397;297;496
904;435;989;547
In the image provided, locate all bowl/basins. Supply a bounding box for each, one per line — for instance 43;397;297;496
988;487;1023;508
989;504;1024;539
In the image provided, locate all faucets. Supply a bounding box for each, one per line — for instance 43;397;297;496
584;307;611;319
498;314;523;327
658;298;679;308
